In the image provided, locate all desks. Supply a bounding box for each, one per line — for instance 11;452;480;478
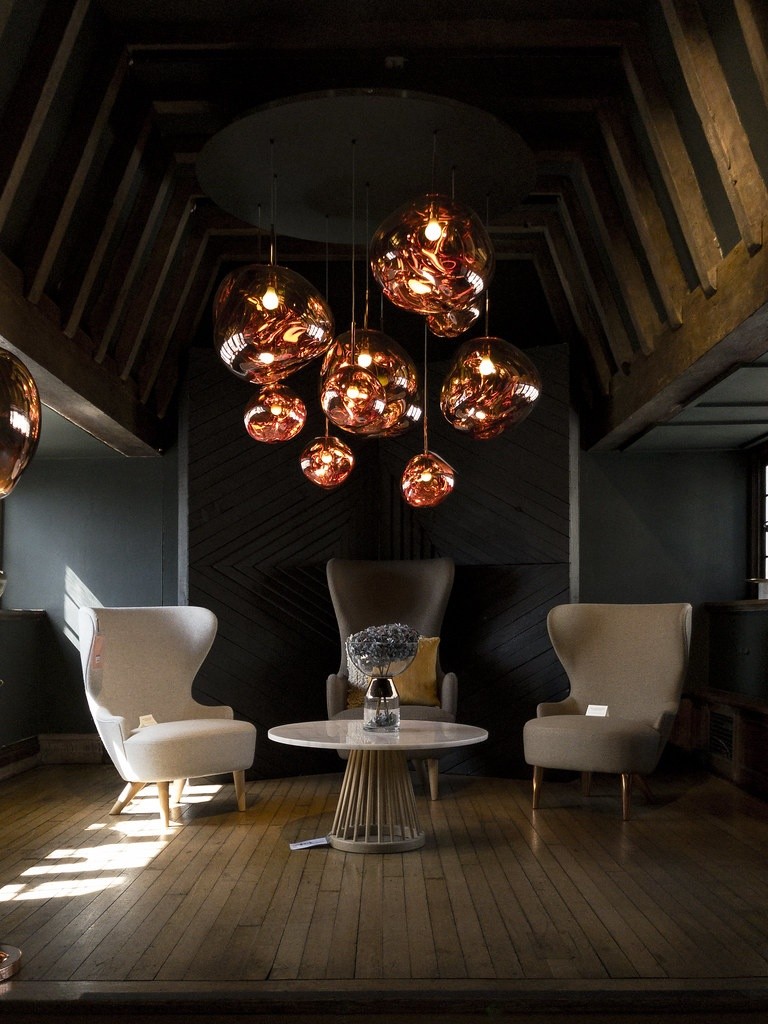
268;719;489;854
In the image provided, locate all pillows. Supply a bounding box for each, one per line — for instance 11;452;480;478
345;634;440;706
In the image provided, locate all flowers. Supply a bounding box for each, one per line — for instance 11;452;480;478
345;624;418;675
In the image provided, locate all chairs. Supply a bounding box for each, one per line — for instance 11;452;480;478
326;557;457;800
78;606;256;828
523;603;692;819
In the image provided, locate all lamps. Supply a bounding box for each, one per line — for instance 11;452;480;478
197;84;542;507
0;347;42;502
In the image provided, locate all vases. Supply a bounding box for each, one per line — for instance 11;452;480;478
347;643;418;733
